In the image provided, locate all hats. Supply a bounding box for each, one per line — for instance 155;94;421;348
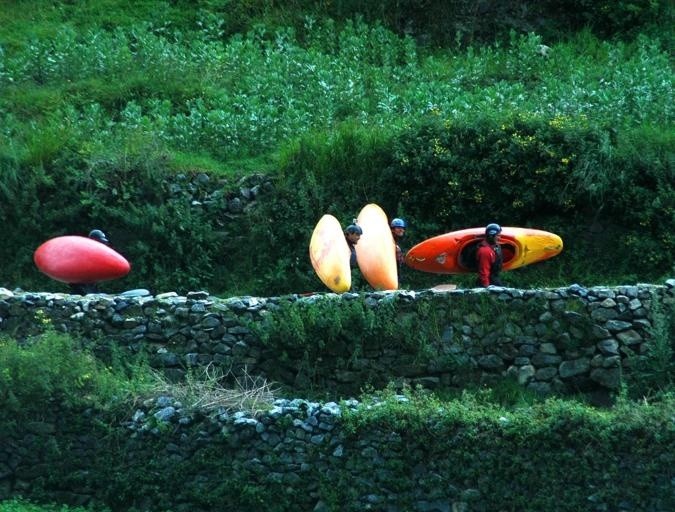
89;229;109;242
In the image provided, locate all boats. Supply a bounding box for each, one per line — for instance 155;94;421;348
309;214;352;292
33;235;132;285
354;203;398;292
407;225;563;275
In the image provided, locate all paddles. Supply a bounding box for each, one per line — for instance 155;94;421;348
121;289;150;297
433;284;457;290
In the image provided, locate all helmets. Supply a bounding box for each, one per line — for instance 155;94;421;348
390;218;406;228
345;223;362;234
486;223;501;236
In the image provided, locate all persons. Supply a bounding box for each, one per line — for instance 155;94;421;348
345;224;363;266
68;230;109;296
389;218;409;289
472;222;504;288
